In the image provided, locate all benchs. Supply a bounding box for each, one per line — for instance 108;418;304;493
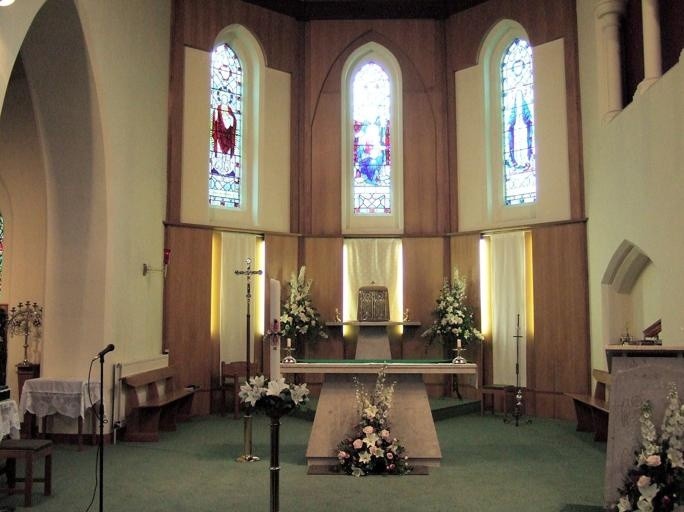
563;369;611;440
122;367;200;442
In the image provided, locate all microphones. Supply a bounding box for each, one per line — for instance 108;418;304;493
92;343;115;361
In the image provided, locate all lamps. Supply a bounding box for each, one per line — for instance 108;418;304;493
143;247;171;278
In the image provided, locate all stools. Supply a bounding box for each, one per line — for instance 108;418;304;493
0;438;52;506
480;384;515;417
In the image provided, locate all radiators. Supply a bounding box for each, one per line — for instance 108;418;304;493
114;353;169;430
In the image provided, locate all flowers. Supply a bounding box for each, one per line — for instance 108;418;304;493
237;374;311;414
609;385;684;512
278;264;330;340
421;265;484;352
334;361;414;477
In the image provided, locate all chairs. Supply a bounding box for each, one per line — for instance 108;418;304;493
221;359;260;419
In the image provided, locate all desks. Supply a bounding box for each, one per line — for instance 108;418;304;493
1;399;20;495
19;377;100;451
279;362;477;476
325;321;422;360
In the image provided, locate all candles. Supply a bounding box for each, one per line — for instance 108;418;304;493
269;278;280;383
457;339;462;347
287;338;291;347
